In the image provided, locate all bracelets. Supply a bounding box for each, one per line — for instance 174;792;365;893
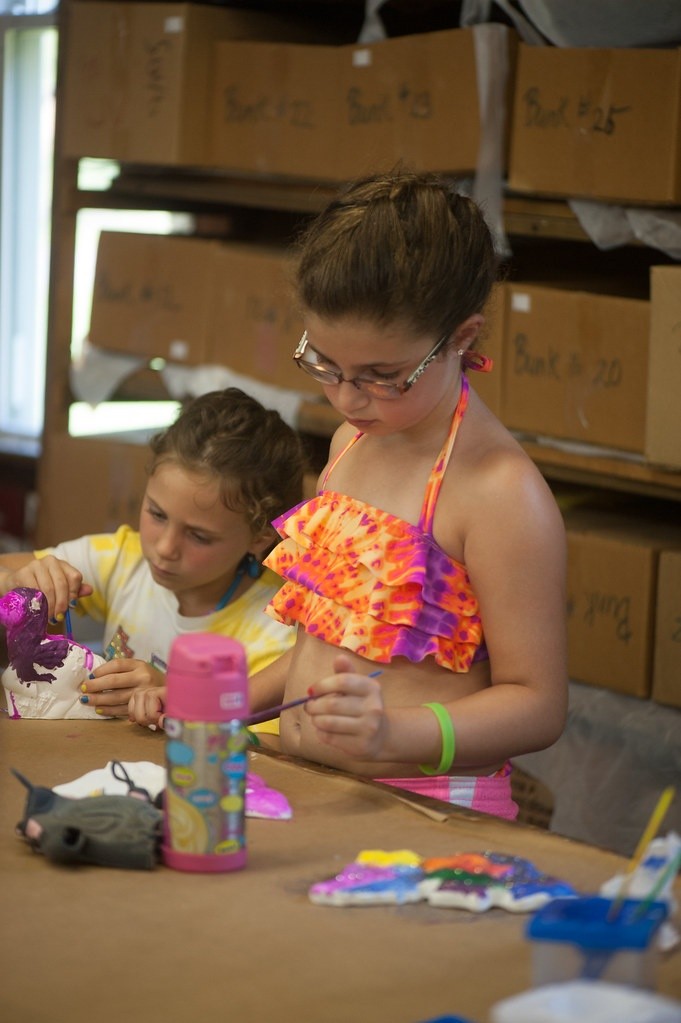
418;703;455;776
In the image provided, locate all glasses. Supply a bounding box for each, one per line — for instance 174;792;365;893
292;326;451;399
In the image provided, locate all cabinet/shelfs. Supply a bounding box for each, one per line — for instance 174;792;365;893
36;3;681;764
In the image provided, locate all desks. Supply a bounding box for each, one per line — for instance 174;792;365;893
0;689;681;1023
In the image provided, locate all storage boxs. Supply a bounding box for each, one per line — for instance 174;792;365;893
85;229;680;470
503;731;679;863
57;0;681;214
46;433;681;705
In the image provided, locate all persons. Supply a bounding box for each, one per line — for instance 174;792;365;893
0;387;304;738
127;177;570;821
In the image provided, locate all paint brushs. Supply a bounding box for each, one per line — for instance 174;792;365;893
246;670;382;725
64;605;75;642
632;850;681;929
607;784;676;925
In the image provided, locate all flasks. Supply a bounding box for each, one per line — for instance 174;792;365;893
161;635;246;873
